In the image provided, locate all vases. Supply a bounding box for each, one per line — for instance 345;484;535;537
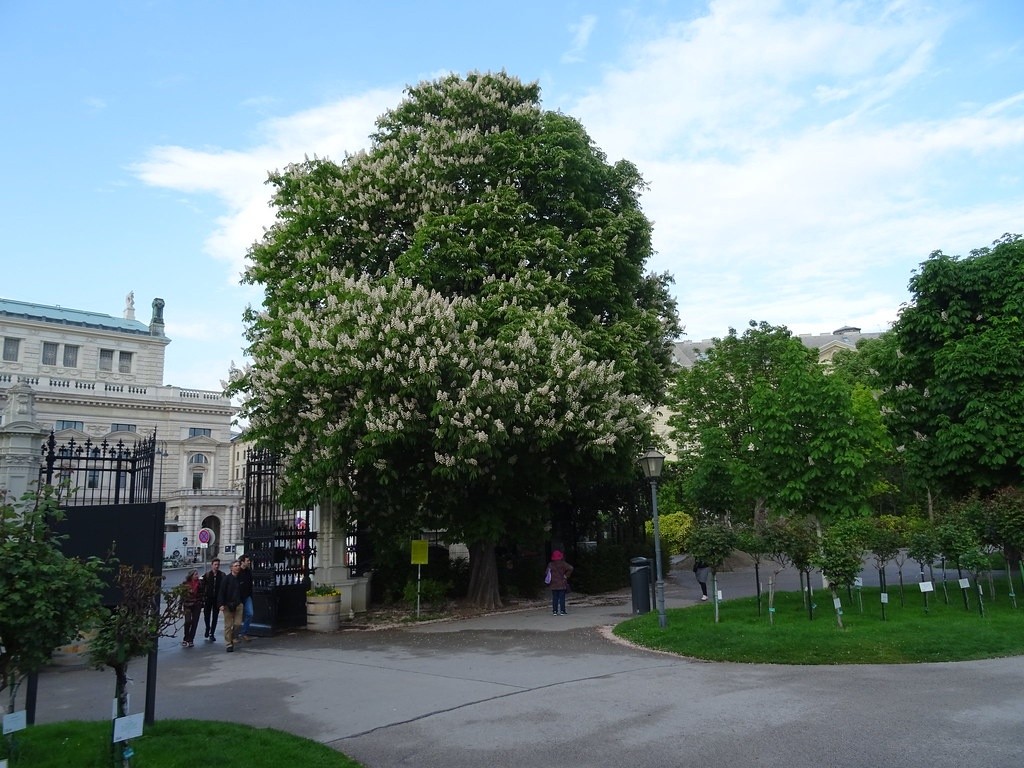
306;595;341;632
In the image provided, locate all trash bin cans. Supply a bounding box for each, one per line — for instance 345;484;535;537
630;557;652;615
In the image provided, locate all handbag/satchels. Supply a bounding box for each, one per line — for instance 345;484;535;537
693;563;698;572
545;569;551;584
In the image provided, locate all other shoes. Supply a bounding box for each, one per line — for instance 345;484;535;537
210;635;216;641
205;627;210;637
701;595;708;600
561;611;568;615
188;641;194;647
182;640;187;646
233;637;241;643
226;644;233;649
241;636;250;641
553;611;557;616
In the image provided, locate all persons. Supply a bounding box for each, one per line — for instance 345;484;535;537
177;555;254;652
693;555;709;600
546;551;573;616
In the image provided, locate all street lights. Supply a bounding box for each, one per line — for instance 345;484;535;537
637;448;668;631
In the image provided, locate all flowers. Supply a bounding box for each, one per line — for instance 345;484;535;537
304;581;341;597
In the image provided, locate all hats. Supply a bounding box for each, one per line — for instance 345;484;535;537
552;550;563;560
